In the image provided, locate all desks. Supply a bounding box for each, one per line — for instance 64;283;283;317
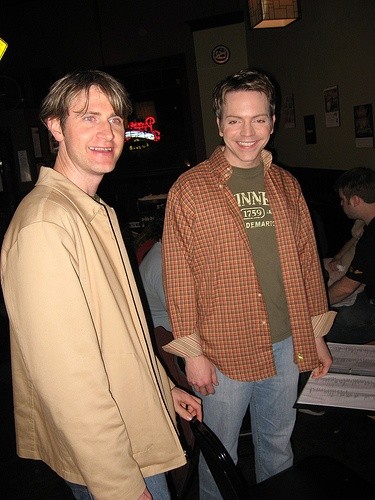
137;195;167;221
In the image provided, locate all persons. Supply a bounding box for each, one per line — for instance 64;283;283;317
0;71;202;500
138;224;186;376
162;70;337;500
297;167;375;416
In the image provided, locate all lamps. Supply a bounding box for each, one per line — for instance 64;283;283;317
249;0;298;30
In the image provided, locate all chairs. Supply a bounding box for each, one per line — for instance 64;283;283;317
188;416;375;500
